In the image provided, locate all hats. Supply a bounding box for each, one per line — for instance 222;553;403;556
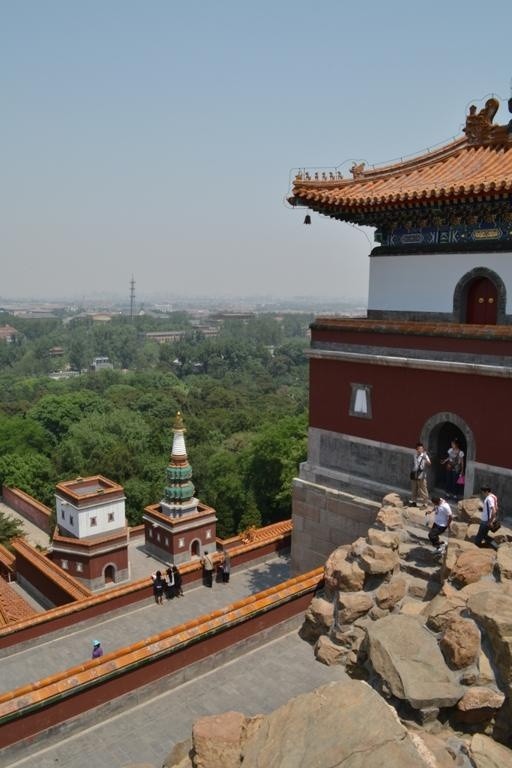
93;640;100;646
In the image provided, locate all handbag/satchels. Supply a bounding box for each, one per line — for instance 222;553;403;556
457;475;464;484
447;463;452;471
490;520;501;532
410;470;422;479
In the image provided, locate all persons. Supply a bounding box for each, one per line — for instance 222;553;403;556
218;551;233;582
473;484;501;549
164;568;175;599
152;570;163;604
201;550;214;586
172;566;184;597
91;639;104;658
408;444;433;510
440;438;465;501
425;496;452;554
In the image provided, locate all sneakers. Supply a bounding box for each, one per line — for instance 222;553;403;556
430;547;442;554
439;541;448;547
